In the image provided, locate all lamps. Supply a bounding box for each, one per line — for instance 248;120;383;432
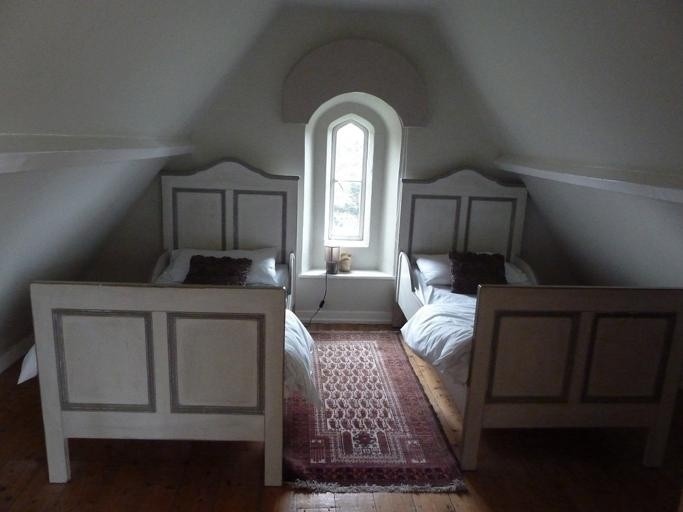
325;245;340;274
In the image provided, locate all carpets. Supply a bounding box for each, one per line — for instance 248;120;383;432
282;329;465;492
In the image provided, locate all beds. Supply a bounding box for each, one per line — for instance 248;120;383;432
29;157;302;487
394;168;683;471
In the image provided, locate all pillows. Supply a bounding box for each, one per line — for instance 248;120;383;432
154;246;280;287
412;251;528;295
